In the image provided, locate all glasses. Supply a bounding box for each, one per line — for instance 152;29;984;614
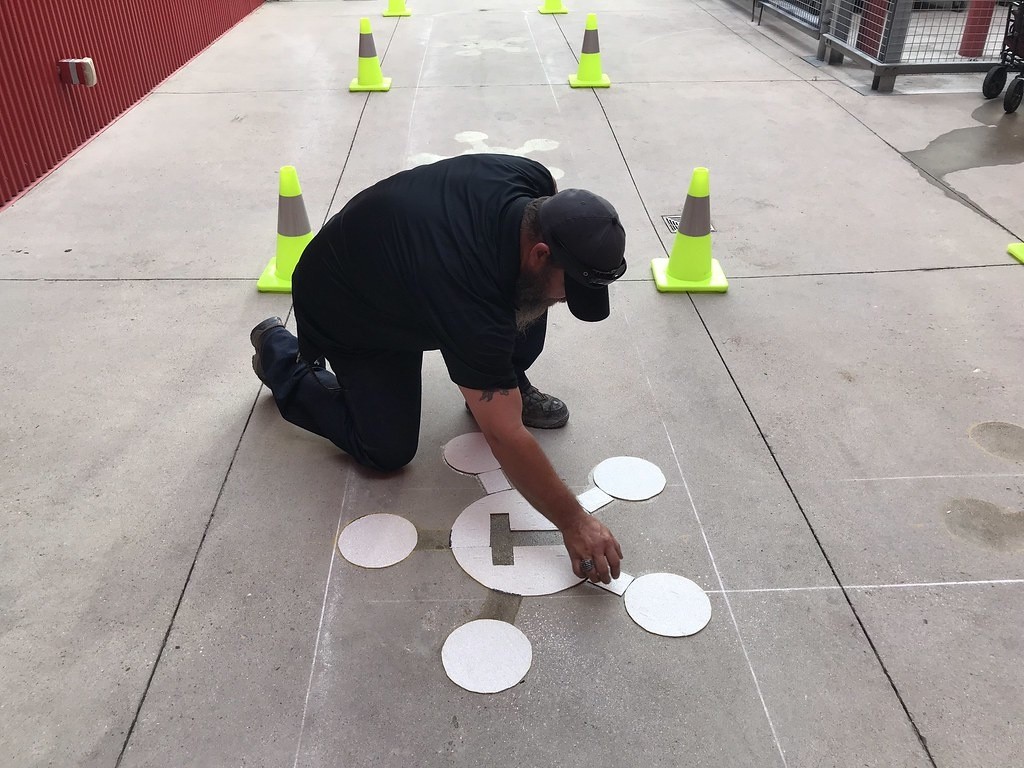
580;258;627;289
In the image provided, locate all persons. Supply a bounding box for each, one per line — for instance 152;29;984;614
250;153;629;585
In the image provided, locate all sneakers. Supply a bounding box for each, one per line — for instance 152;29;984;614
251;315;284;390
465;385;569;429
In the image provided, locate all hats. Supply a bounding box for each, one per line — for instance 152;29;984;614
540;188;626;322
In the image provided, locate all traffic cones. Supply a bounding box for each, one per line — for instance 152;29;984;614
568;12;612;89
347;17;392;92
255;165;315;293
538;0;569;14
382;0;413;17
651;167;729;293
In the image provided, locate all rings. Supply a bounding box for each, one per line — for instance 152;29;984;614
580;559;595;571
